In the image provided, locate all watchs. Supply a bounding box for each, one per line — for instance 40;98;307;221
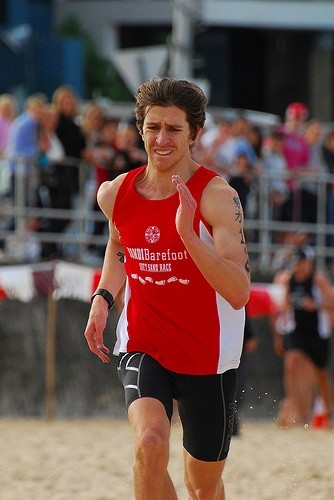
90;289;116;310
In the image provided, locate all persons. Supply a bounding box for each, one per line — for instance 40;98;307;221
275;254;334;427
0;80;334;282
84;78;253;500
